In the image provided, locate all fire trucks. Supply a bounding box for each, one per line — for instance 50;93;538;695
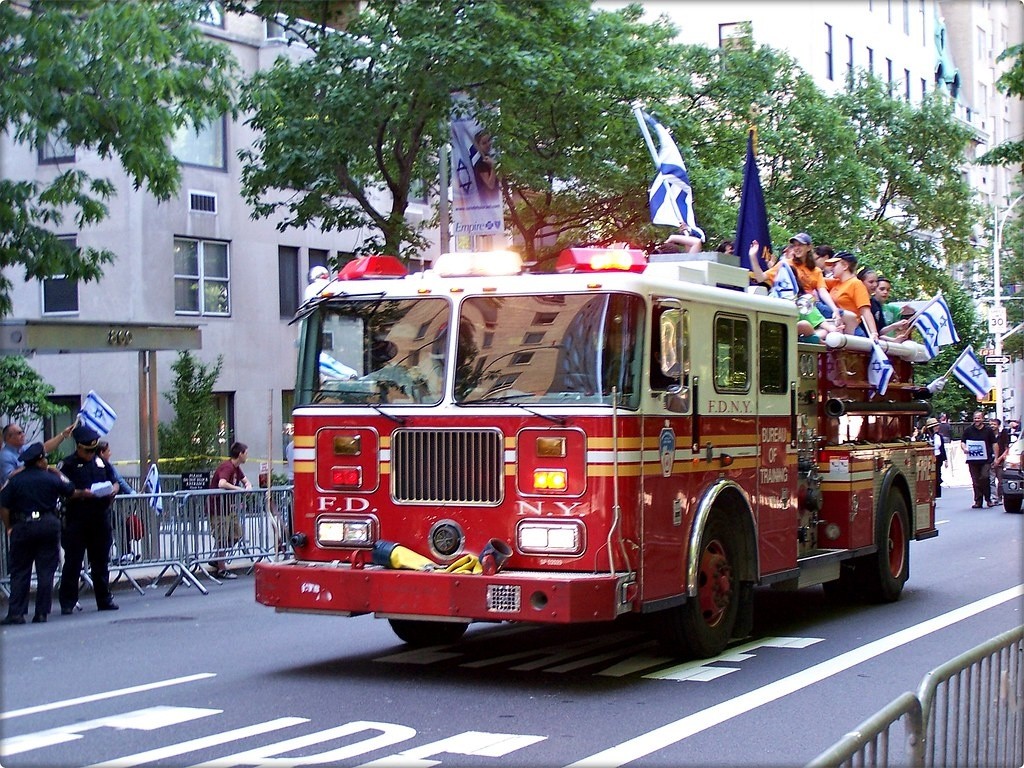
254;246;939;662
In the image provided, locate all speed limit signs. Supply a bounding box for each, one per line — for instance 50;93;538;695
988;307;1006;333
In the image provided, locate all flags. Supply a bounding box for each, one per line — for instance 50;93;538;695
76;390;117;437
147;464;162;517
952;349;992;397
643;112;705;242
734;142;773;272
914;297;961;358
868;344;894;399
774;263;799;299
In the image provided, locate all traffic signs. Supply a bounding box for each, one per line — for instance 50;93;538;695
984;356;1013;365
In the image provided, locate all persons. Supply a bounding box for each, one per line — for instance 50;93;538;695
655;223;915;344
602;313;674;391
359;356;442;394
281;440;294;542
204;442;251;579
913;377;1019;508
0;423;74;625
57;425;119;615
473;130;499;203
97;440;136;557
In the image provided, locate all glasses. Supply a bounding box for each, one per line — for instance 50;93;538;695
8;431;24;437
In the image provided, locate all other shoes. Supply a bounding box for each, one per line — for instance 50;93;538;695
98;602;119;610
208;561;218;567
991;498;998;503
61;607;72;614
32;613;47;622
972;502;982;508
998;500;1003;505
987;501;993;507
216;569;237;579
0;614;25;624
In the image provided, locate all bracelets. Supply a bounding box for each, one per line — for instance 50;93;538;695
61;433;66;439
873;333;878;335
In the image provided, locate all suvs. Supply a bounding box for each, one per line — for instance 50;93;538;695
1001;440;1024;515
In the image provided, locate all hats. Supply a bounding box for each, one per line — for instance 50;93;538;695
857;268;869;278
926;418;941;429
824;251;857;266
789;232;811;245
878;277;891;282
73;426;100;452
21;442;50;463
